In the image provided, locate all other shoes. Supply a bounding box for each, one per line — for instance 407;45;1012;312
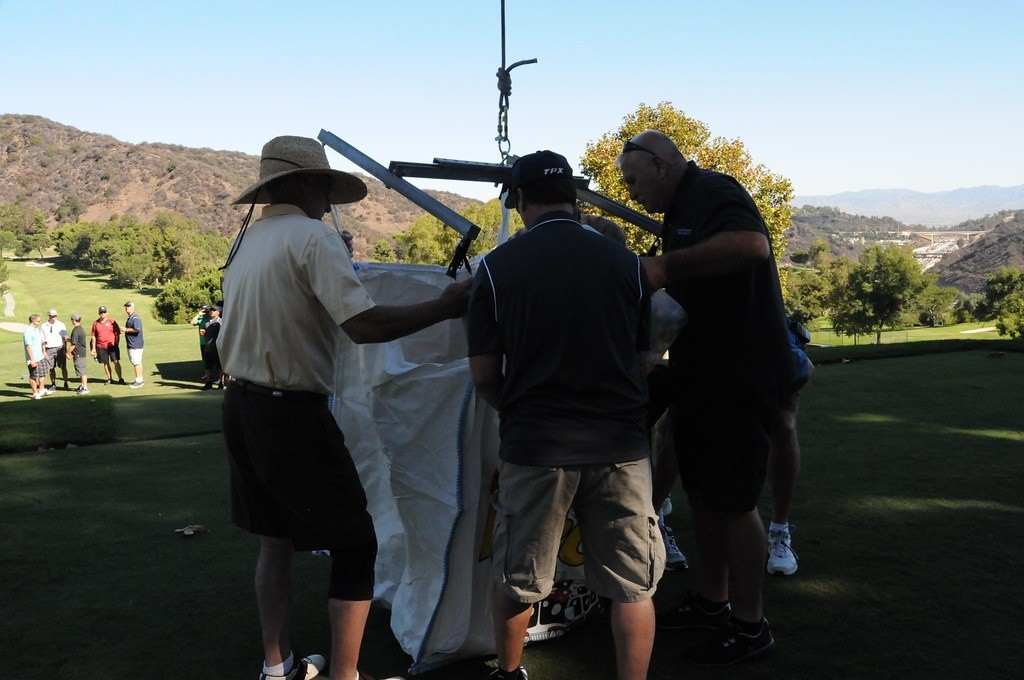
63;384;70;389
32;389;53;400
75;385;90;395
118;378;127;385
201;383;212;391
130;381;145;388
105;379;113;385
49;384;57;391
218;383;224;390
490;665;528;680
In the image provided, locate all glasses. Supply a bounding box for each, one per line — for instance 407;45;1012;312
622;141;657;157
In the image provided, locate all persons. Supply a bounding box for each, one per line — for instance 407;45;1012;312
464;151;668;680
615;129;795;667
190;300;229;390
216;136;472;680
653;317;815;576
22;300;146;400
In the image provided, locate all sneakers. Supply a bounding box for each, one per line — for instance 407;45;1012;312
260;654;328;680
767;523;800;576
664;528;690;572
655;596;731;632
688;622;779;665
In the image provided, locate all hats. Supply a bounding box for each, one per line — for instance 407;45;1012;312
99;306;107;312
505;150;572;210
209;305;219;311
47;310;58;316
70;313;81;321
230;135;368;204
124;302;134;306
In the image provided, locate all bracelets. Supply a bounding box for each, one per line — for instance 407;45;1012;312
127;327;128;332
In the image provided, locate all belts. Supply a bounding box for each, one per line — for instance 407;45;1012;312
234;378;327;405
